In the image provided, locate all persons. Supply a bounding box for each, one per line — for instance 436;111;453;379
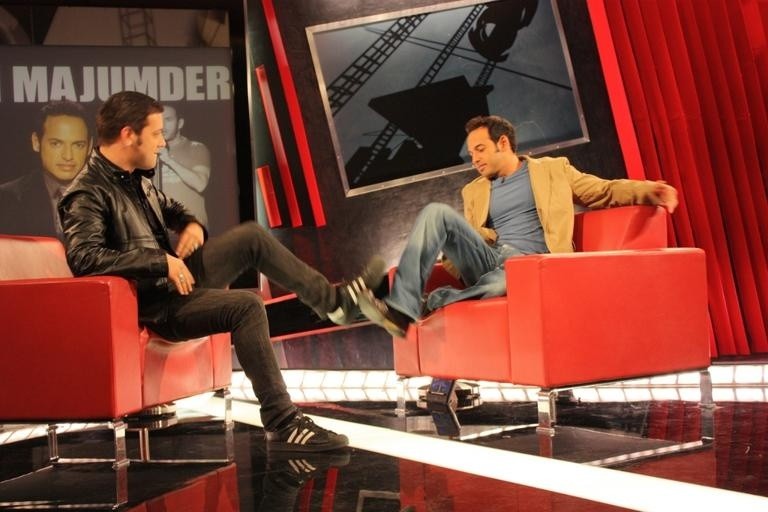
57;89;387;451
355;115;681;340
254;437;351;512
149;102;215;237
0;100;96;238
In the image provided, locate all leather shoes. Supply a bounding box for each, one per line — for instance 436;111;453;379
358;292;409;337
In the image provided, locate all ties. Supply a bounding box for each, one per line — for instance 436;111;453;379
55;188;66;237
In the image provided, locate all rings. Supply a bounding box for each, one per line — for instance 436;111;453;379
194;242;199;249
178;274;185;282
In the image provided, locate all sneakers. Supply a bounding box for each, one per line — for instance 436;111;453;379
326;254;387;329
265;409;349;452
266;450;351;486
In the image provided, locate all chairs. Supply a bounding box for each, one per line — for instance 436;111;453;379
0;234;236;511
387;204;715;469
